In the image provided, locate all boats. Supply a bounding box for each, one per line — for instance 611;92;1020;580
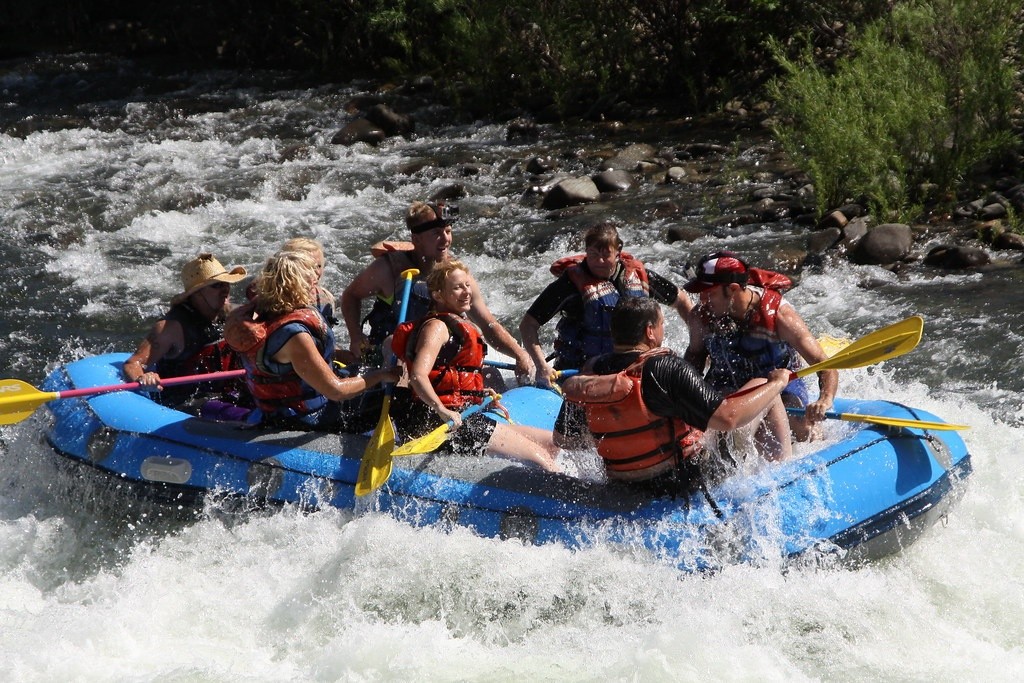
30;350;975;578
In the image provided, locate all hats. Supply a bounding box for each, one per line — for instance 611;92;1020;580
683;252;750;294
170;253;247;305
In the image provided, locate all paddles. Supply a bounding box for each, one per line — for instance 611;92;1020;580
0;368;247;428
389;393;501;456
483;359;580;377
721;315;924;399
786;403;970;431
352;267;422;498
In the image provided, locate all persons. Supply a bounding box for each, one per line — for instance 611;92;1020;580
121;200;404;431
340;198;845;487
